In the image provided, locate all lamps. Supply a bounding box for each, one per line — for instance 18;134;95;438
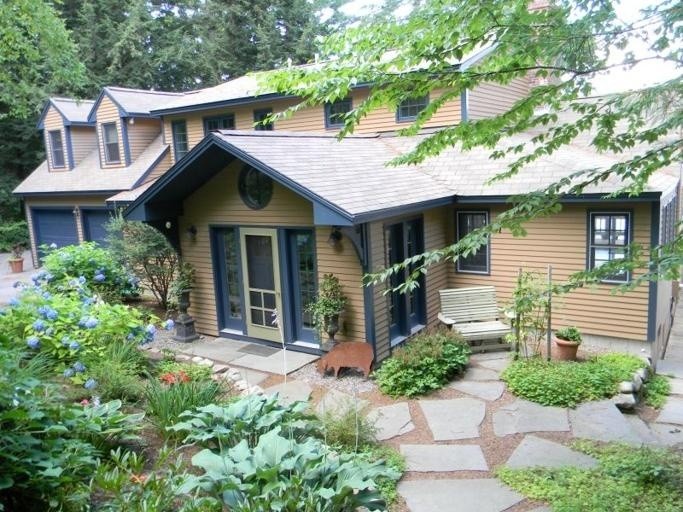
326;226;342;246
185;226;196;240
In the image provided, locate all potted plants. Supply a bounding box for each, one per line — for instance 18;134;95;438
553;326;582;360
0;220;31;273
303;274;348;351
174;262;200;343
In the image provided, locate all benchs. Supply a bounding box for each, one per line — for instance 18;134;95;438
438;285;516;353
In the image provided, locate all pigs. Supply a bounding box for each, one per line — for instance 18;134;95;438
317;341;374;380
